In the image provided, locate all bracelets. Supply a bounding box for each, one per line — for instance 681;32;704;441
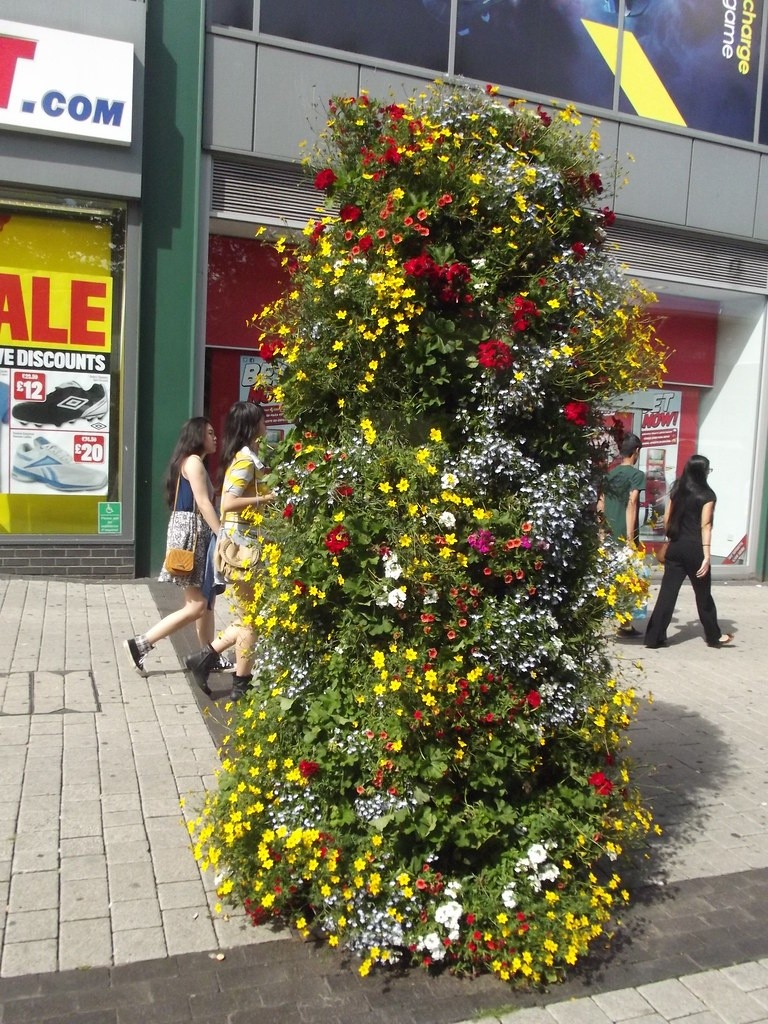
702;545;710;546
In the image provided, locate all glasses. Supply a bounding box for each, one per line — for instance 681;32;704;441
707;469;713;474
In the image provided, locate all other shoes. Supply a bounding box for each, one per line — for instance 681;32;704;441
709;633;735;647
647;640;669;649
124;636;155;677
616;627;645;640
213;653;234;670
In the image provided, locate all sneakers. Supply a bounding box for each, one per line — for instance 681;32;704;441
13;438;107;493
11;381;110;428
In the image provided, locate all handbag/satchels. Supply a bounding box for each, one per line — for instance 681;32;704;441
165;549;197;577
624;561;648;619
215;537;260;583
658;543;671;563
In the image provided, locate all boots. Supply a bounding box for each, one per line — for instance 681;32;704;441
183;642;220;696
230;672;253;701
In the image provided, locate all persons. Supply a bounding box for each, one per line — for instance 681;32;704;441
644;455;733;648
596;432;646;638
123;416;239;677
184;402;275;699
